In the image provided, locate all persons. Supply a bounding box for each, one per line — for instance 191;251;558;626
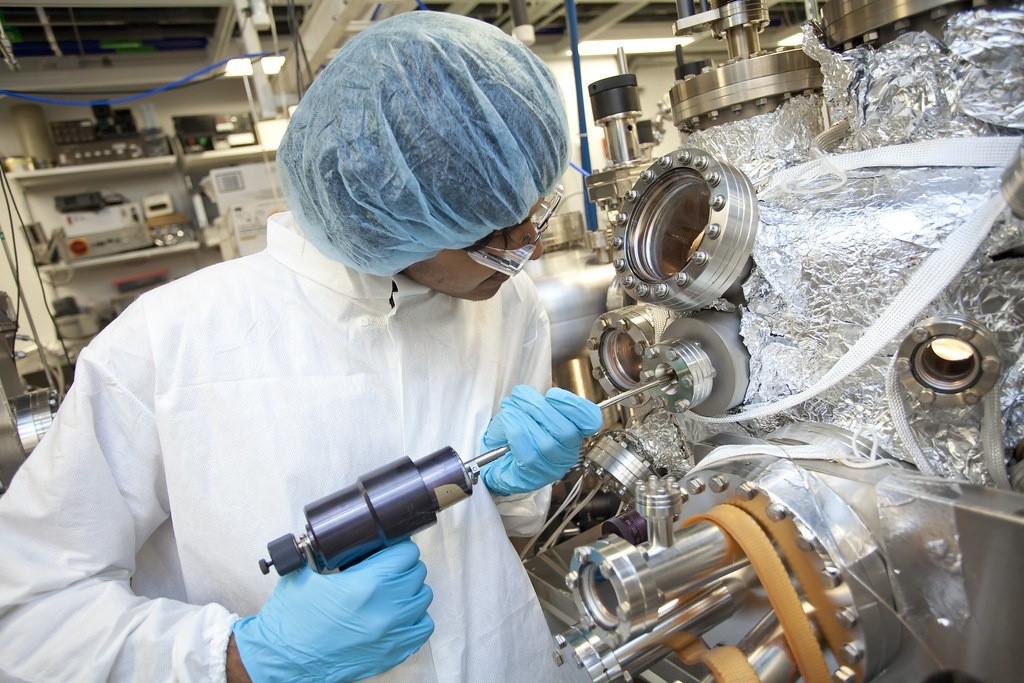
0;10;601;682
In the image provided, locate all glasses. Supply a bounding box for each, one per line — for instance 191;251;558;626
465;183;564;278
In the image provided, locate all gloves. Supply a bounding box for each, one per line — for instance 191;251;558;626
232;539;435;683
479;383;604;496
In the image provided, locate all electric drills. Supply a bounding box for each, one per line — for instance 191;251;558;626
257;372;674;577
